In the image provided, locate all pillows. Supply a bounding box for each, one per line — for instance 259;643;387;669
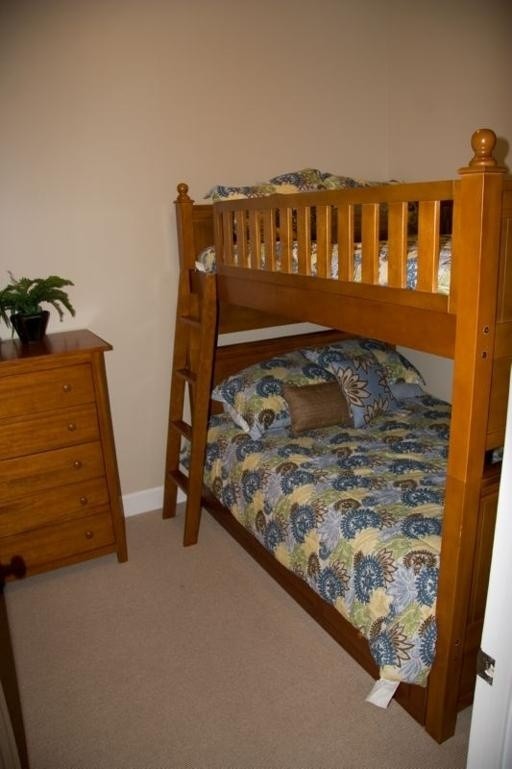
304;339;427;408
331;356;400;427
212;353;338;442
205;168;409;222
280;384;351;436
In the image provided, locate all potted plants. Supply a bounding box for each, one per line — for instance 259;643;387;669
0;270;75;344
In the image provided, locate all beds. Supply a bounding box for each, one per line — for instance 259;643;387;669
164;128;511;744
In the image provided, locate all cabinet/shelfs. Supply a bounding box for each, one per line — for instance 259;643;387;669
0;331;128;581
